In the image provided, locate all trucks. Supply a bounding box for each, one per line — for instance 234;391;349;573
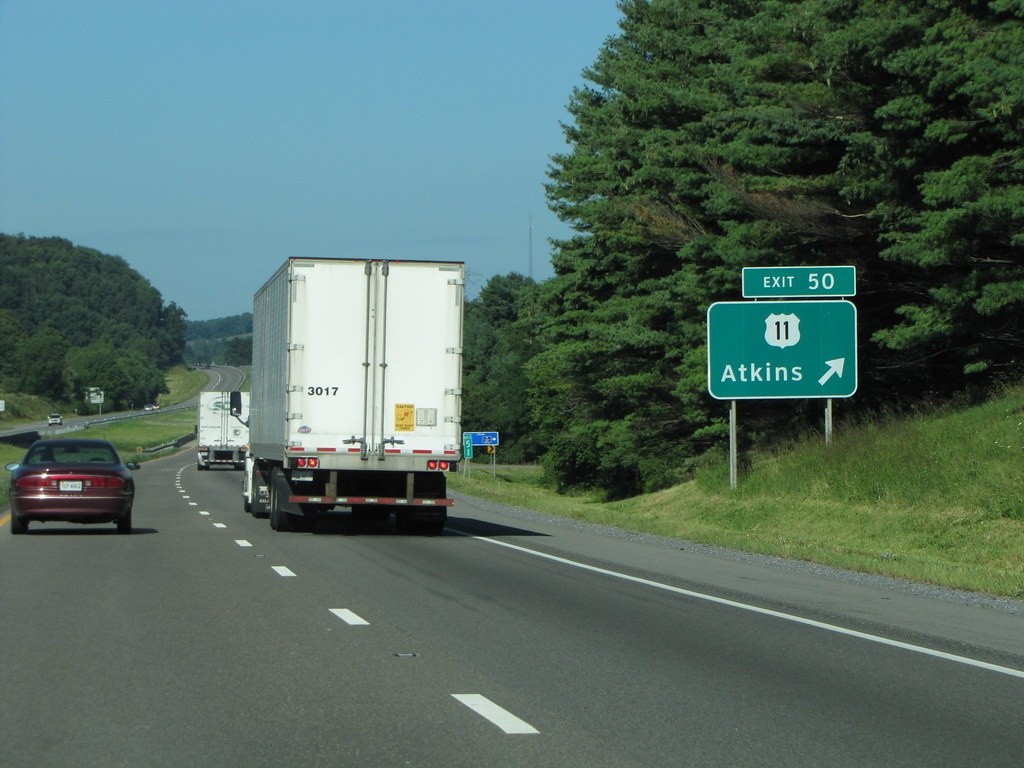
243;256;464;535
197;391;251;470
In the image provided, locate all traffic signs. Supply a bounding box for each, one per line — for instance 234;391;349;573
707;301;858;399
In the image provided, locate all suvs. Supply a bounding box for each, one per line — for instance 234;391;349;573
48;414;63;425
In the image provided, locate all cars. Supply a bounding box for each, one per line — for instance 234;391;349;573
6;438;141;535
143;403;160;411
